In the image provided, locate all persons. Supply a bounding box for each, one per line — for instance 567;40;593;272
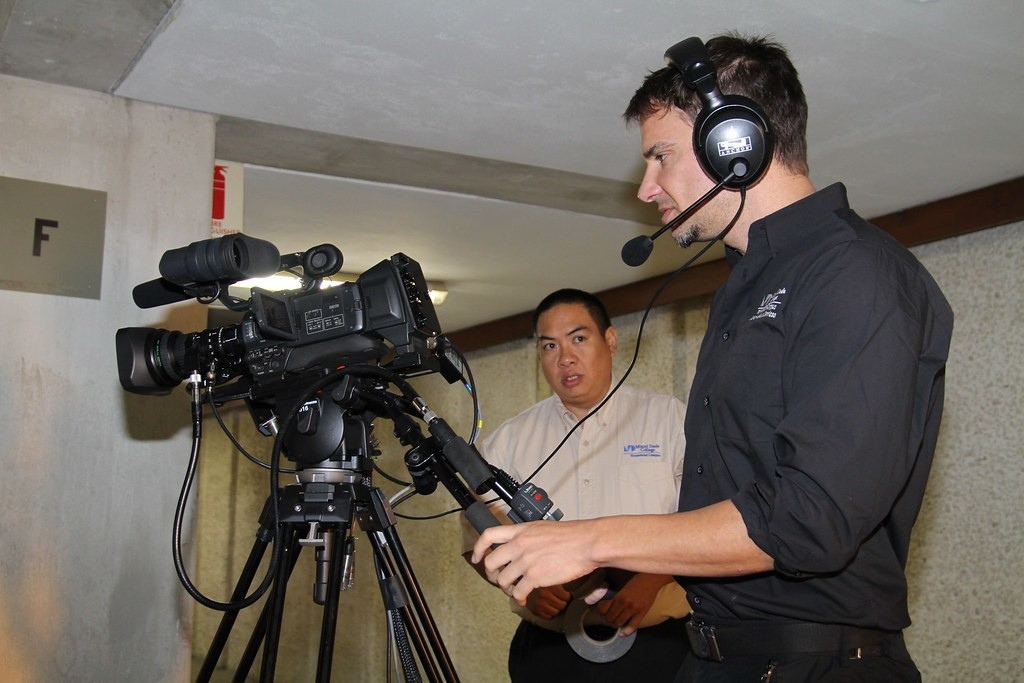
459;287;689;683
471;31;955;683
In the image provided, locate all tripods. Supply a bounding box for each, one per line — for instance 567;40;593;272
197;388;564;683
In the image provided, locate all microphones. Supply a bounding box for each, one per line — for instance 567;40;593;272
622;159;750;266
131;277;196;307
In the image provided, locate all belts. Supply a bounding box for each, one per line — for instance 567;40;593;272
684;618;896;661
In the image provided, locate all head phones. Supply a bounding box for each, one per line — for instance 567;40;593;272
664;35;775;192
